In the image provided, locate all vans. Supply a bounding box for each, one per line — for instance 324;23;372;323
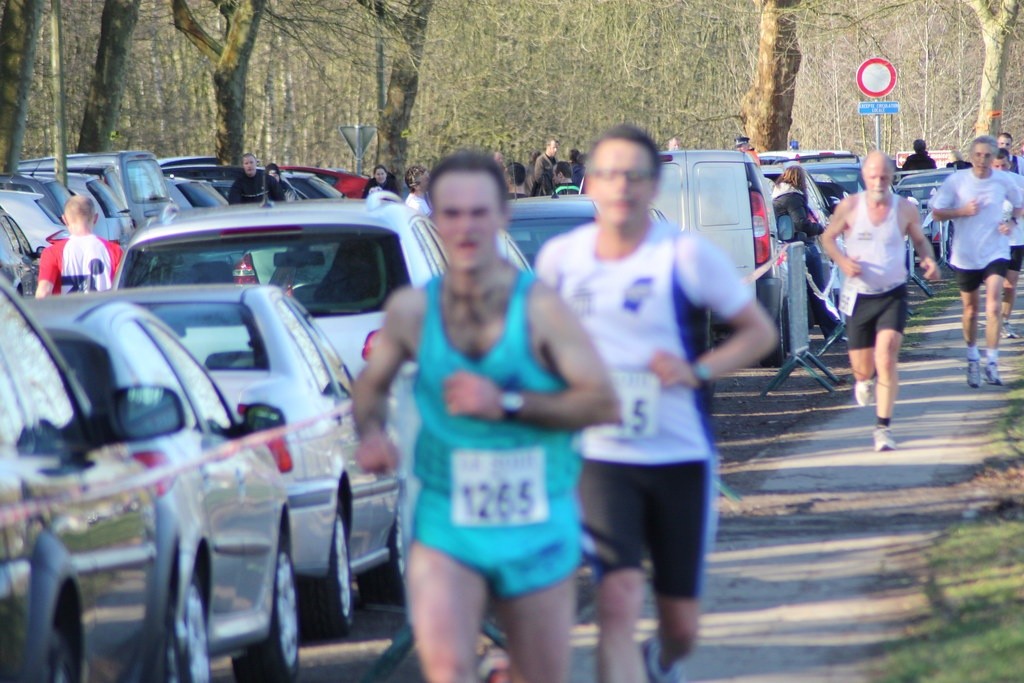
644;149;791;367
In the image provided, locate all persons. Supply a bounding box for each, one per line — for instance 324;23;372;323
734;137;760;165
405;166;432;216
536;124;779;683
931;135;1024;388
35;195;125;297
228;152;286;206
772;166;841;339
265;163;298;200
997;133;1024;176
352;150;622;683
821;150;939;451
991;148;1024;338
362;165;399;201
668;138;682;150
901;139;937;179
494;139;586;199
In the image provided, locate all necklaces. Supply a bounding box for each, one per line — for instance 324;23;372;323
946;150;973;170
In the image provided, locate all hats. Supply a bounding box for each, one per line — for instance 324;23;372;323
734;136;750;147
265;163;279;174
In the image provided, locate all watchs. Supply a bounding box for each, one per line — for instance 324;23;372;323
500;390;524;419
693;366;709;387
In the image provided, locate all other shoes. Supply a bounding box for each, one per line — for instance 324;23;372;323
827;336;848;346
477;647;510;683
641;637;686;683
1000;321;1019;338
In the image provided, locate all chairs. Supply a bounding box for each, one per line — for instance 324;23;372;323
313;241;378;303
186;261;233;284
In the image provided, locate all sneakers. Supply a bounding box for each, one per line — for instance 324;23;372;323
873;424;896;452
982;361;1002;386
966;359;980;388
855;379;874;407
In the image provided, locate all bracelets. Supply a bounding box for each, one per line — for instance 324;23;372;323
1011;217;1018;224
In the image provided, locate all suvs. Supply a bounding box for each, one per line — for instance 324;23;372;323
113;191;528;507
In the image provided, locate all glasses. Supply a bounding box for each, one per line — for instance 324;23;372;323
589;167;657;183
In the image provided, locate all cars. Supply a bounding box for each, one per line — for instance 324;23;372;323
502;192;672;281
760;148;956;254
0;153;404;683
